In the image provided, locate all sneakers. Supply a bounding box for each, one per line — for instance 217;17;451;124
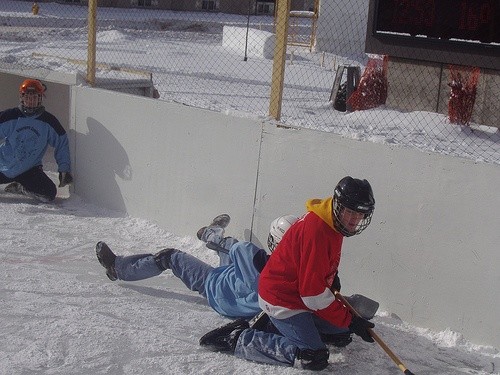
249;311;273;330
200;318;250;353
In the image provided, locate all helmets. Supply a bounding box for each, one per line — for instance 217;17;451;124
267;213;301;252
17;78;47;112
333;175;376;237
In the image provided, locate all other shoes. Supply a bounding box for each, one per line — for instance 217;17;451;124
197;213;230;239
96;240;118;280
4;181;24;193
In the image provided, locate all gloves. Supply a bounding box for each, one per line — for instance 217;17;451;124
331;270;341;291
349;310;374;342
59;171;73;186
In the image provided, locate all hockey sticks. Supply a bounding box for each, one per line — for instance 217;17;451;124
333;290;415;375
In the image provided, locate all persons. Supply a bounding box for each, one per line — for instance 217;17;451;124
199;175;375;371
96;214;298;321
0;79;73;202
